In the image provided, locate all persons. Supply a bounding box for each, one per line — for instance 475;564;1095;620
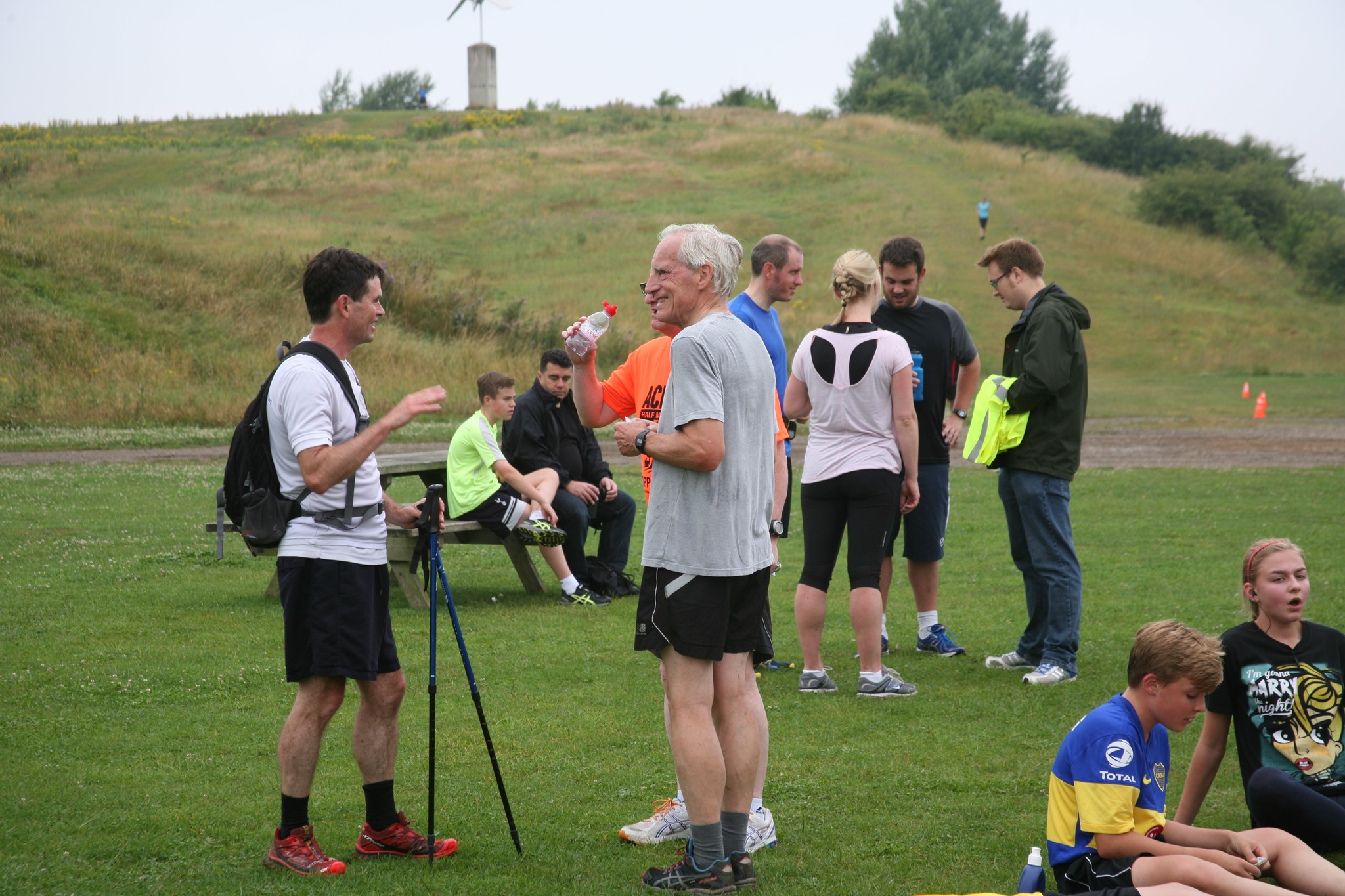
261;246;460;878
500;348;641;598
561;278;778;855
783;250;918;698
1172;536;1345;854
445;371;612;606
1046;620;1345;896
641;222;777;896
727;233;804;670
976;198;991;240
854;235;980;657
977;238;1091;687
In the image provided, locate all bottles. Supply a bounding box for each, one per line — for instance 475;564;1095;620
1017;847;1046;893
910;343;924;401
566;300;617;357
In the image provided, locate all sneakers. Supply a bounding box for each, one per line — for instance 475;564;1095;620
916;623;965;657
743;806;778;854
799;665;838;693
754;671;760;678
641;836;737;896
263;825;346;877
856;664;918;699
560;584;612;606
1022;663;1077;684
619;798;692;846
515;518;567;547
353;811;458;858
728;850;756;888
753;659;795;670
855;635;888;658
985;651;1038;670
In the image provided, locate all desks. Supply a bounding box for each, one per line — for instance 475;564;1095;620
265;450;547;607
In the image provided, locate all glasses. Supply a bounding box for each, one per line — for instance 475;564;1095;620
989;267;1022;289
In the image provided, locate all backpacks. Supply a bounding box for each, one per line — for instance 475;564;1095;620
223;340;369;556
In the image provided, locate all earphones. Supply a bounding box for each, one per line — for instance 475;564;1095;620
1252;591;1257;596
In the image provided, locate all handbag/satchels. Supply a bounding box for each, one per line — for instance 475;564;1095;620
587;555;640;597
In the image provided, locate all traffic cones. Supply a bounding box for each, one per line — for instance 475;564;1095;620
1259;391;1268;409
1242;381;1250;398
1254;396;1265;418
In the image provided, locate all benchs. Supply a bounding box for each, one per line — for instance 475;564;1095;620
204;520;486;536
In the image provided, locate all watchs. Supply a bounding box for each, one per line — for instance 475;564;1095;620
768;519;784;538
951;408;969;421
635;429;650;458
